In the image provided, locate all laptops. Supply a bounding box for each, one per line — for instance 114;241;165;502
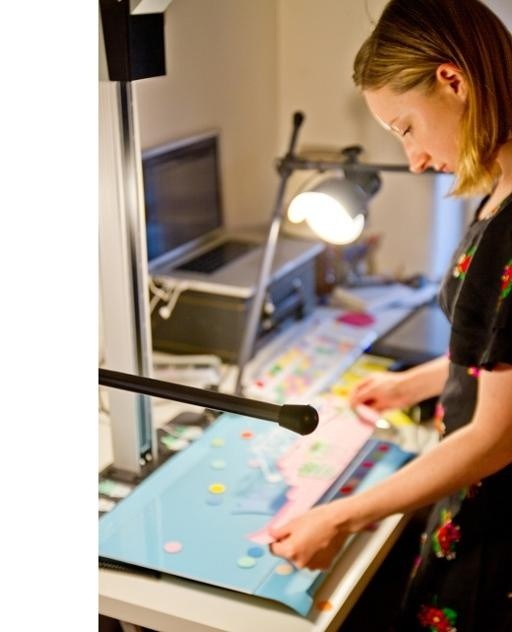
142;127;326;300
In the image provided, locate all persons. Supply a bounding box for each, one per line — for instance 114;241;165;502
263;0;512;631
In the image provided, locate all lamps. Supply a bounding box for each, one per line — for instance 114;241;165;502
236;110;465;395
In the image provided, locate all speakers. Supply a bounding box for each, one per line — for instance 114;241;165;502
98;0;167;81
